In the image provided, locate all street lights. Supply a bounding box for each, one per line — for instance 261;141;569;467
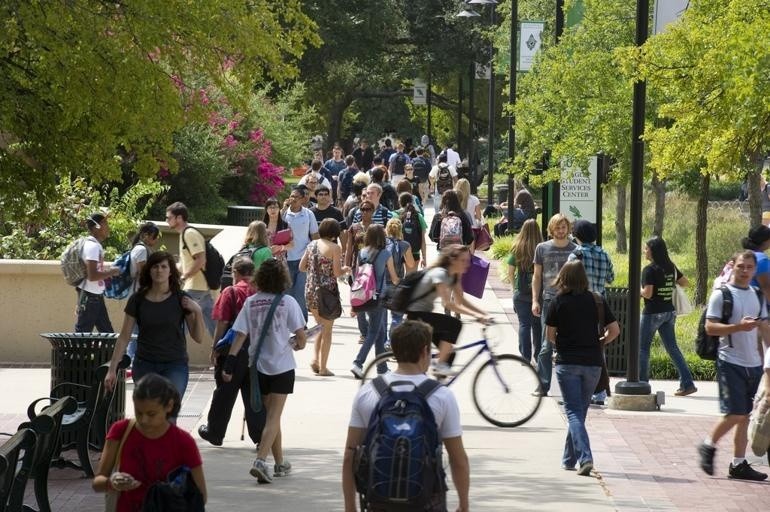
432;0;519;235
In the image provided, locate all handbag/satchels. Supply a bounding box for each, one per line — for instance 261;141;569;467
214;329;244;358
317;287;341;319
750;390;770;454
673;267;692;315
473;223;493;250
249;365;262;412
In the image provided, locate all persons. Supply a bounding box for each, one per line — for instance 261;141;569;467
223;257;306;485
545;260;620;475
91;374;207;511
70;131;622;395
698;224;770;481
104;253;206;424
197;257;256;447
639;236;697;396
343;319;469;512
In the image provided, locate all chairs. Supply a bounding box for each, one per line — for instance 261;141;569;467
0;425;38;510
28;353;133;479
0;396;80;511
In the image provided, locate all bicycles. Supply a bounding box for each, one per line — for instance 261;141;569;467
361;315;543;428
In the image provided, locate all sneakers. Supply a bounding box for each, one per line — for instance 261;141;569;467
700;444;767;481
351;365;367;379
250;461;291;484
675;386;697;396
578;461;593;474
534;385;547;397
311;363;334;376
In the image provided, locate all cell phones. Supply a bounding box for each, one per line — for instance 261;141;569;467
113;476;131;486
754;317;761;320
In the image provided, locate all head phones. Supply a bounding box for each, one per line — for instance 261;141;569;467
86;215;101;229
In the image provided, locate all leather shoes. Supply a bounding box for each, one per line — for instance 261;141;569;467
199;425;222;445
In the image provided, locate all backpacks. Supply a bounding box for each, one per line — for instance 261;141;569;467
220;245;266;292
104;243;147;300
414;159;427;183
61;237;96;286
387;267;446;311
402;220;421;252
438;210;463;247
182;227;224;291
351;248;385;311
352;376;442;508
437;164;453;194
696;285;733;359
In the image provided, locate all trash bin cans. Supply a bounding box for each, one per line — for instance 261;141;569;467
39;332;138;449
227;206;266;226
601;287;632;376
